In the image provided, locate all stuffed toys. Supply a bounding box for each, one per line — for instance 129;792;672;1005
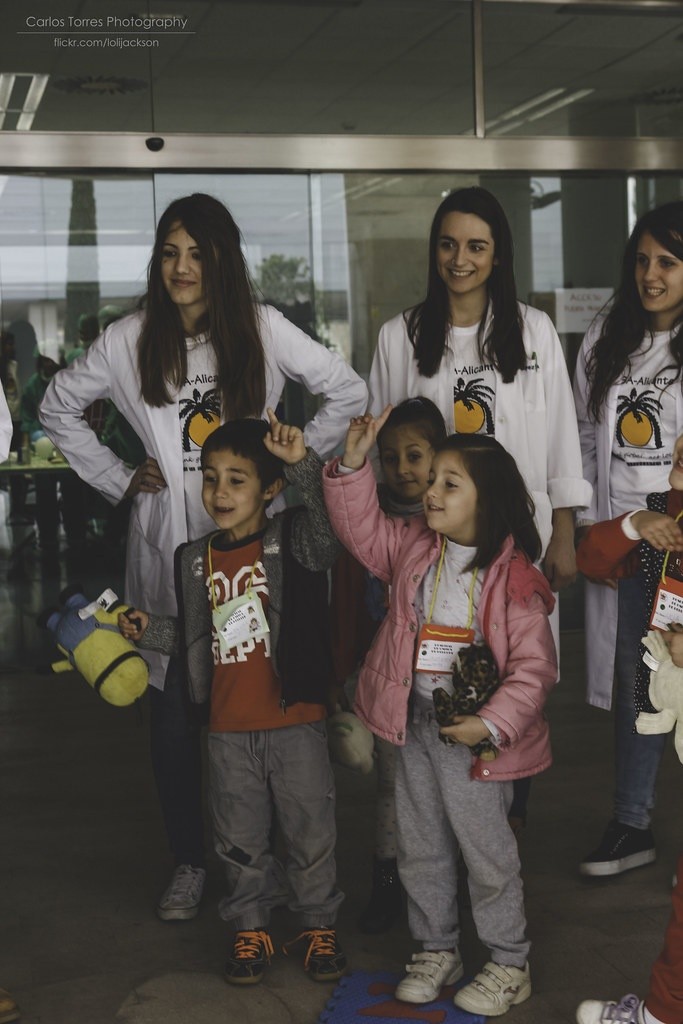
433;645;503;762
39;581;149;707
635;628;683;764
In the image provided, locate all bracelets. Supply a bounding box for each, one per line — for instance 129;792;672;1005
338;462;354;474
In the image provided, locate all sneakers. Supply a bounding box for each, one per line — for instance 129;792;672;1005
394;949;464;1004
282;926;347;980
579;819;658;876
224;928;274;984
453;959;531;1017
156;863;207;921
576;993;647;1024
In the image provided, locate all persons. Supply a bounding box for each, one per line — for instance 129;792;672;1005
117;409;348;986
38;192;367;921
0;381;12;463
0;307;146;574
575;622;683;1024
321;403;558;1015
578;434;683;734
358;185;593;839
575;200;683;881
326;396;450;930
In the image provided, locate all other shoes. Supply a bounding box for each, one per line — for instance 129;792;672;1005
357;858;403;935
